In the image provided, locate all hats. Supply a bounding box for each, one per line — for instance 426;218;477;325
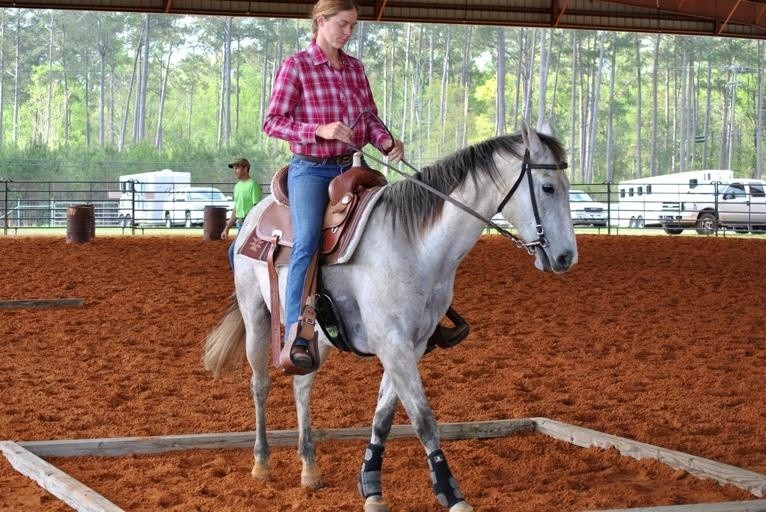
228;159;250;168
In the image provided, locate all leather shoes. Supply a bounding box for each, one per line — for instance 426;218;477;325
291;344;312;369
423;324;468;354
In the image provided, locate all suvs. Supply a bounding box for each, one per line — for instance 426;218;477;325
660;178;766;234
163;187;235;229
568;190;609;227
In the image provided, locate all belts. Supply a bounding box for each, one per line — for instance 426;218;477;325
294;153;353;166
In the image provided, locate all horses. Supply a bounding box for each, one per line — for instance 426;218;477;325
199;114;579;512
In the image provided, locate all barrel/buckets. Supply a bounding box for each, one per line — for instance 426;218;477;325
202;205;227;241
65;206;91;245
71;203;96;241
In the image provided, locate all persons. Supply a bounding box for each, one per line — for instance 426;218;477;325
260;0;471;375
219;158;265;300
